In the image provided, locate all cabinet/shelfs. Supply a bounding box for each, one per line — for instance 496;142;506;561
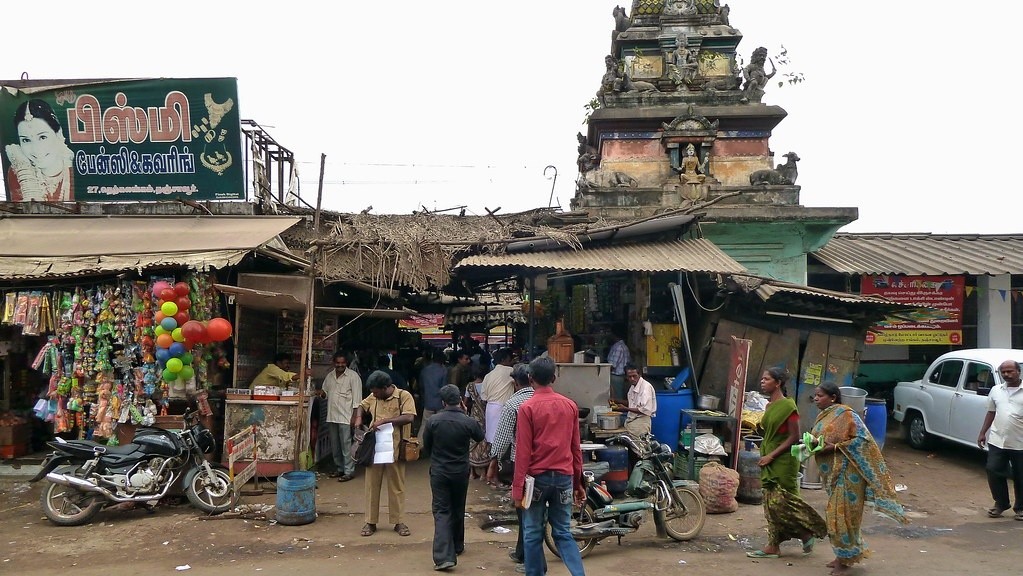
276;316;335;365
674;409;737;481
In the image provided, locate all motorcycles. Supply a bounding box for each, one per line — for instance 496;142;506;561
27;407;241;526
544;428;706;560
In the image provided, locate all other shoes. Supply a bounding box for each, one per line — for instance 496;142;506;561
988;502;1011;517
434;562;454;570
485;481;512;490
1014;511;1023;520
455;545;464;555
479;476;487;481
470;474;476;479
328;471;344;477
338;474;354;481
509;550;520;562
515;564;547;573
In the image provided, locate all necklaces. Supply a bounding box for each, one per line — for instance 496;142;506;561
204;91;234;129
191;143;232;176
30;160;70;202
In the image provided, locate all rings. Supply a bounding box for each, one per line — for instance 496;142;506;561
192;117;228;143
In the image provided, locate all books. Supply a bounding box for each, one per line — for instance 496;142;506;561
521;473;535;510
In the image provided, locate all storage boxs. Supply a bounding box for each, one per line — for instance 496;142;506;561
673;424;729;481
0;422;33;460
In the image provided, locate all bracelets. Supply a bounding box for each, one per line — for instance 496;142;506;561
10;161;43;202
834;444;837;452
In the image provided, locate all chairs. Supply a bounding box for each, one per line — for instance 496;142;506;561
977;370;992;384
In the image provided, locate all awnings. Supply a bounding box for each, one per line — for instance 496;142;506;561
213;283;413;349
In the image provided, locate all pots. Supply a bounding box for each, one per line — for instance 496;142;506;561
546;334;574;362
696;393;720;409
597;415;621;428
599;416;617;429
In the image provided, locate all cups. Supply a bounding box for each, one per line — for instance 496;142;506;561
595;357;600;364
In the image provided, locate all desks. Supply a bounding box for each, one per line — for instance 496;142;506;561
589;422;627;445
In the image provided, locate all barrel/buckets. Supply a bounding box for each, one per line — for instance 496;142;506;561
800;437;821;483
651;389;694;451
864;397;887;451
839;386;868;420
276;471;315;525
600;445;628;492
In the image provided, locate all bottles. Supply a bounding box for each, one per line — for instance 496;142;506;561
237;323;266;381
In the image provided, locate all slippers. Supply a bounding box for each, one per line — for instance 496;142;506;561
361;523;376;536
746;549;780;558
394;524;411;536
802;536;815;556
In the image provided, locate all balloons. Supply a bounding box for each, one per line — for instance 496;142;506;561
153;280;233;382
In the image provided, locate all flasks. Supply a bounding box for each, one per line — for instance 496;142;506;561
671;348;679;366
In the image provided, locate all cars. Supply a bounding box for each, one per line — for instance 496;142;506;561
890;348;1023;472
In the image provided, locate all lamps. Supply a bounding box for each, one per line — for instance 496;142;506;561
462;280;474;295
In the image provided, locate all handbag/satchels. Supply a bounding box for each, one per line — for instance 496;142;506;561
398;438;420;462
351;424;376;467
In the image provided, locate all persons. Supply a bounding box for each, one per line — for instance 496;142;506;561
356;416;361;418
596;32;777;110
747;367;910;576
423;356;589;576
978;359;1023;521
672;143;709;184
607;328;657;498
249;352;314;393
4;99;75;202
367;338;528;490
317;350;417;536
